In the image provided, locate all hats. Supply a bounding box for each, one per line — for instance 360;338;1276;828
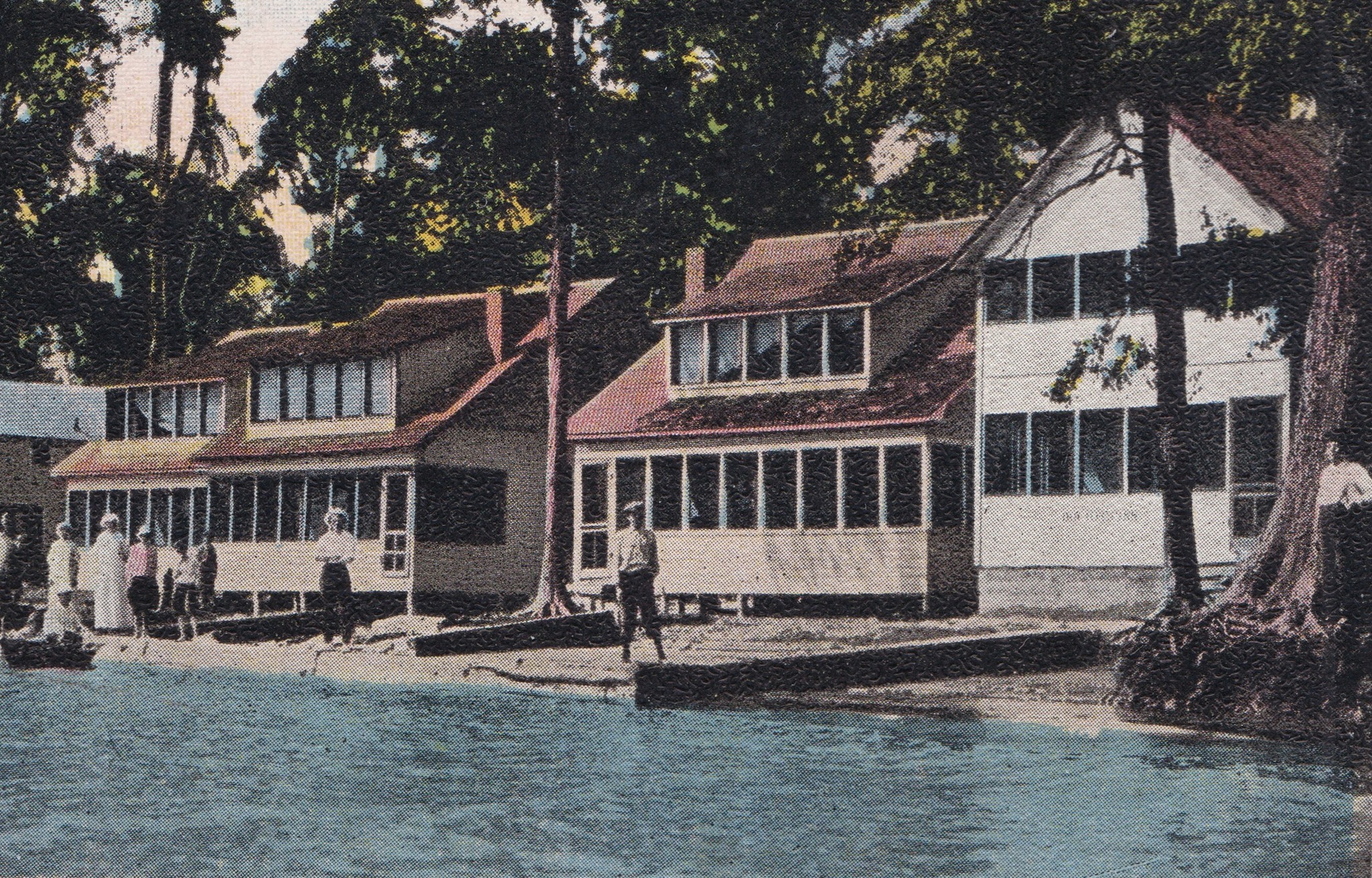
324;507;349;527
621;500;645;512
136;525;153;536
57;521;72;529
100;513;120;527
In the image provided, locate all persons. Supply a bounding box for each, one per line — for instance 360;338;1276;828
610;501;668;665
0;507;218;651
1307;428;1371;632
314;507;358;648
